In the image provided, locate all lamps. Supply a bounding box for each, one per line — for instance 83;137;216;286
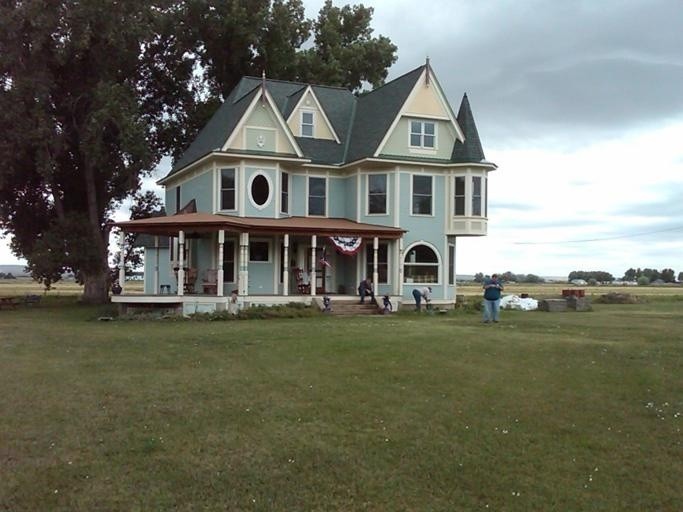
322;298;330;309
382;297;389;308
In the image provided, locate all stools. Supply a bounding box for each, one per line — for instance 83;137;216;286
160;285;170;295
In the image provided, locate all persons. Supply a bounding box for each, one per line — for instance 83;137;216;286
481;273;503;324
357;275;375;303
411;286;432;311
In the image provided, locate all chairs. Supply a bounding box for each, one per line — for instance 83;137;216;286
200;269;217;294
